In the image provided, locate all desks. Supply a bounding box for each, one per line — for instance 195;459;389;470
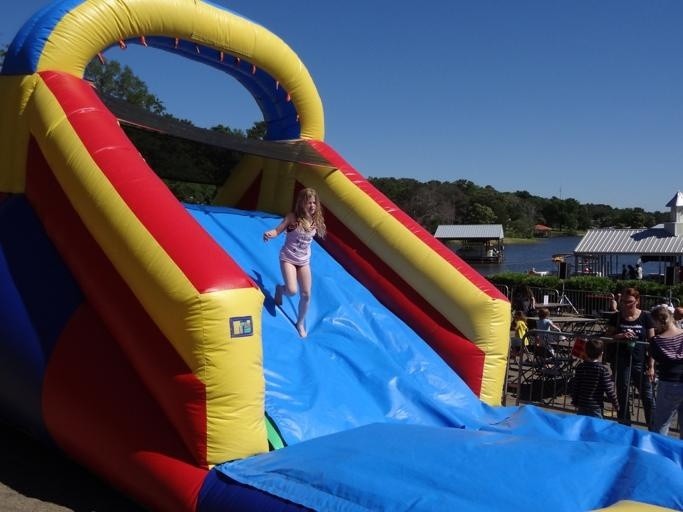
525;303;597;342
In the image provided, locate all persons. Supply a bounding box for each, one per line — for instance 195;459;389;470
584;259;592;272
527;290;537;316
488;242;496;252
265;187;327;339
608;287;683;440
512;312;533;364
636;264;643;280
627;265;638;280
572;340;621;418
535;309;562;367
622;264;627;280
